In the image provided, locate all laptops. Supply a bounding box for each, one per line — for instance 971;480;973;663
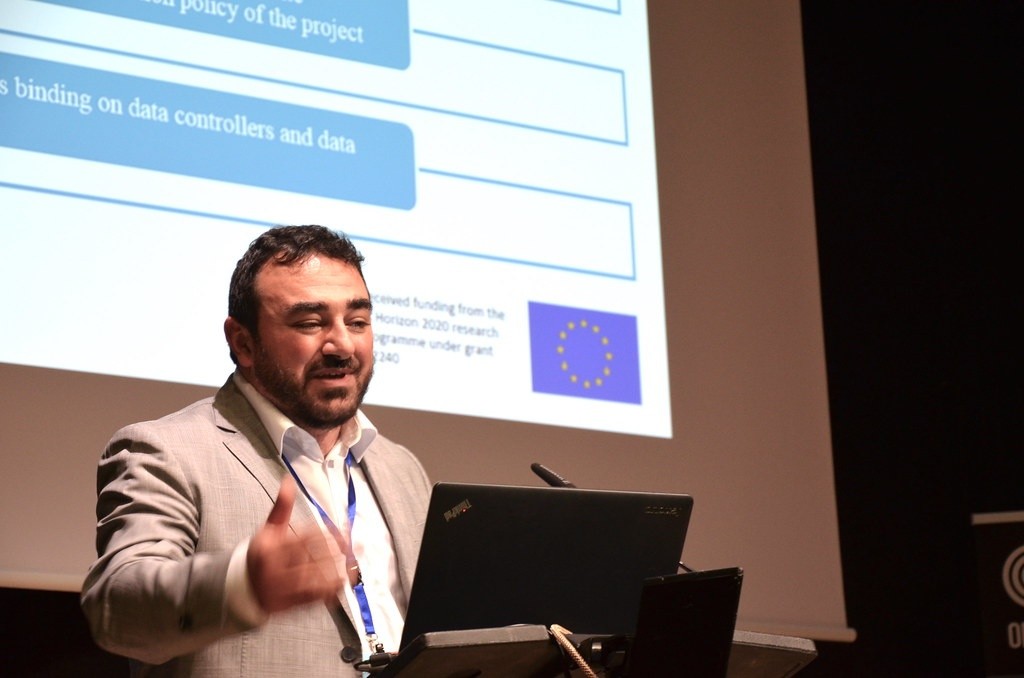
393;483;692;651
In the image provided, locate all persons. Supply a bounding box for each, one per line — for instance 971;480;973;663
80;225;431;678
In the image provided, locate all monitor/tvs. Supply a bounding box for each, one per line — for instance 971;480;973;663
622;567;744;678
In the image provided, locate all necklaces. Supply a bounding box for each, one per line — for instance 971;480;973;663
281;448;379;655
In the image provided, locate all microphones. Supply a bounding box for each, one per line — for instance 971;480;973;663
529;463;693;572
